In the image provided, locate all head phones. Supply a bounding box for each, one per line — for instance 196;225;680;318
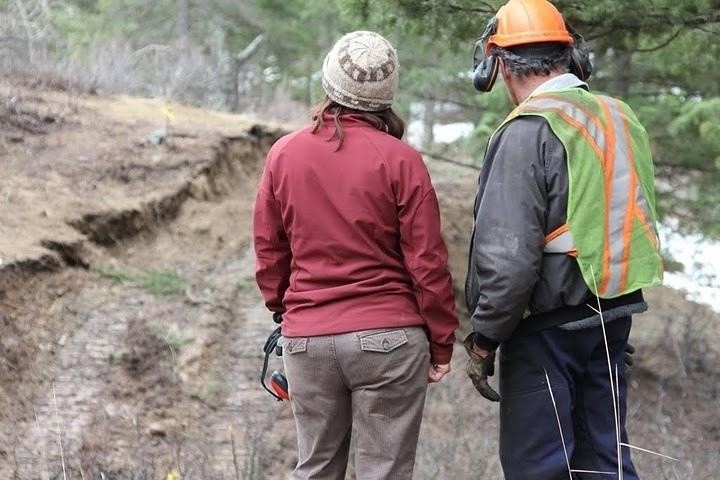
472;17;592;92
261;326;289;402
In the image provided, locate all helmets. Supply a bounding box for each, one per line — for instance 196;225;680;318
484;0;574;54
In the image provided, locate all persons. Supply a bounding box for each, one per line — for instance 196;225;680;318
251;31;461;480
460;0;666;480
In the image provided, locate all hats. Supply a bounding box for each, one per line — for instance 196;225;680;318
321;31;400;112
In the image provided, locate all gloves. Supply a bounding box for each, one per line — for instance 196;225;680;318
462;332;501;402
